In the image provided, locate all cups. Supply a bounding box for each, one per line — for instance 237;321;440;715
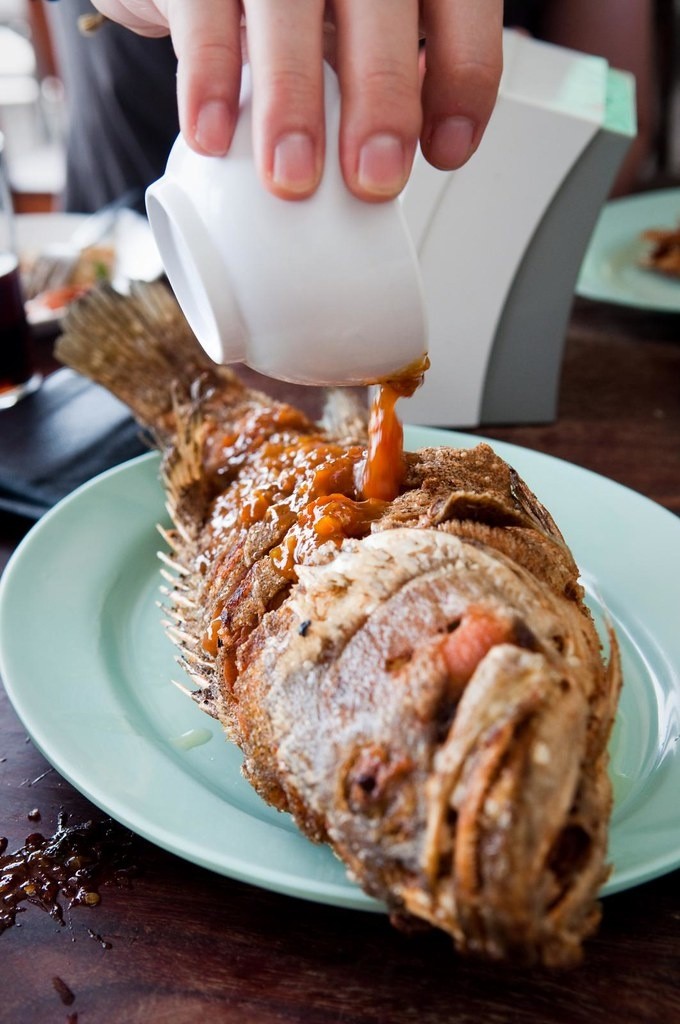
0;177;44;410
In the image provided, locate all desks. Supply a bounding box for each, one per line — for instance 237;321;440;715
0;293;679;1024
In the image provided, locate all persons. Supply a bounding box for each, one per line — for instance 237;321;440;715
42;1;503;223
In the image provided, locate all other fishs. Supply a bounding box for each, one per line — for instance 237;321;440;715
53;279;626;971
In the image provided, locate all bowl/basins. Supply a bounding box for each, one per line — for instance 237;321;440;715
144;55;426;386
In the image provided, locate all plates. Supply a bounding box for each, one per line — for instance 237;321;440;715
0;366;157;519
14;209;166;325
574;186;680;309
0;424;680;912
